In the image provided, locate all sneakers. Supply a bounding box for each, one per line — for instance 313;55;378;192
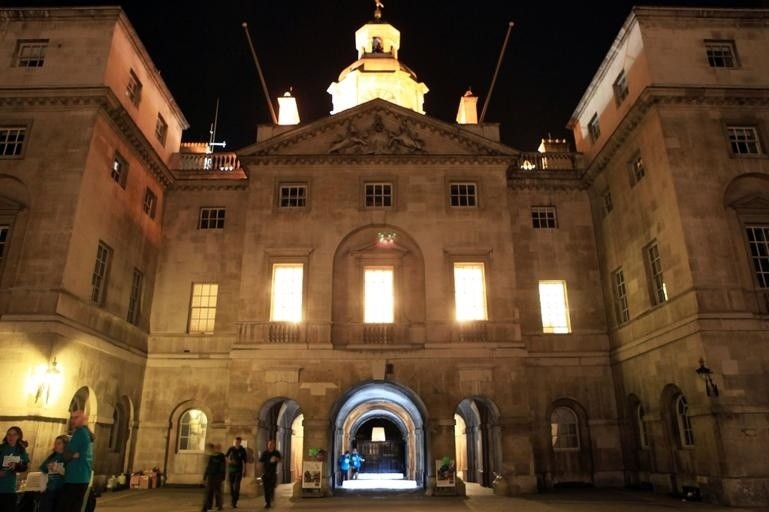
345;477;358;480
201;503;238;512
264;503;271;509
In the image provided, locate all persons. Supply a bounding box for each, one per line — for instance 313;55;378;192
225;436;248;509
258;438;283;510
337;450;350;483
35;434;69;512
63;410;96;511
198;443;228;512
0;425;29;511
350;447;365;480
